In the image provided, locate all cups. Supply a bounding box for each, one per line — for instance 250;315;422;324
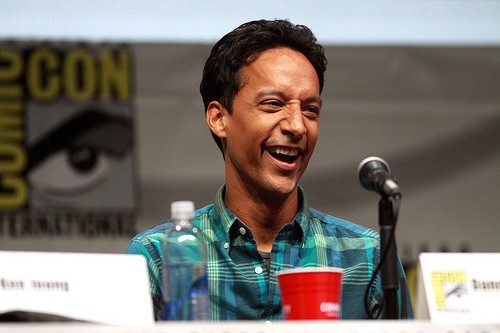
274;267;343;321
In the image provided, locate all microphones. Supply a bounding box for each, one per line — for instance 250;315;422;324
357;156;401;200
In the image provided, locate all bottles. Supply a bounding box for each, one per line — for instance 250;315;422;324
162;201;211;322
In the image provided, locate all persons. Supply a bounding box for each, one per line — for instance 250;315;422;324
125;19;414;321
